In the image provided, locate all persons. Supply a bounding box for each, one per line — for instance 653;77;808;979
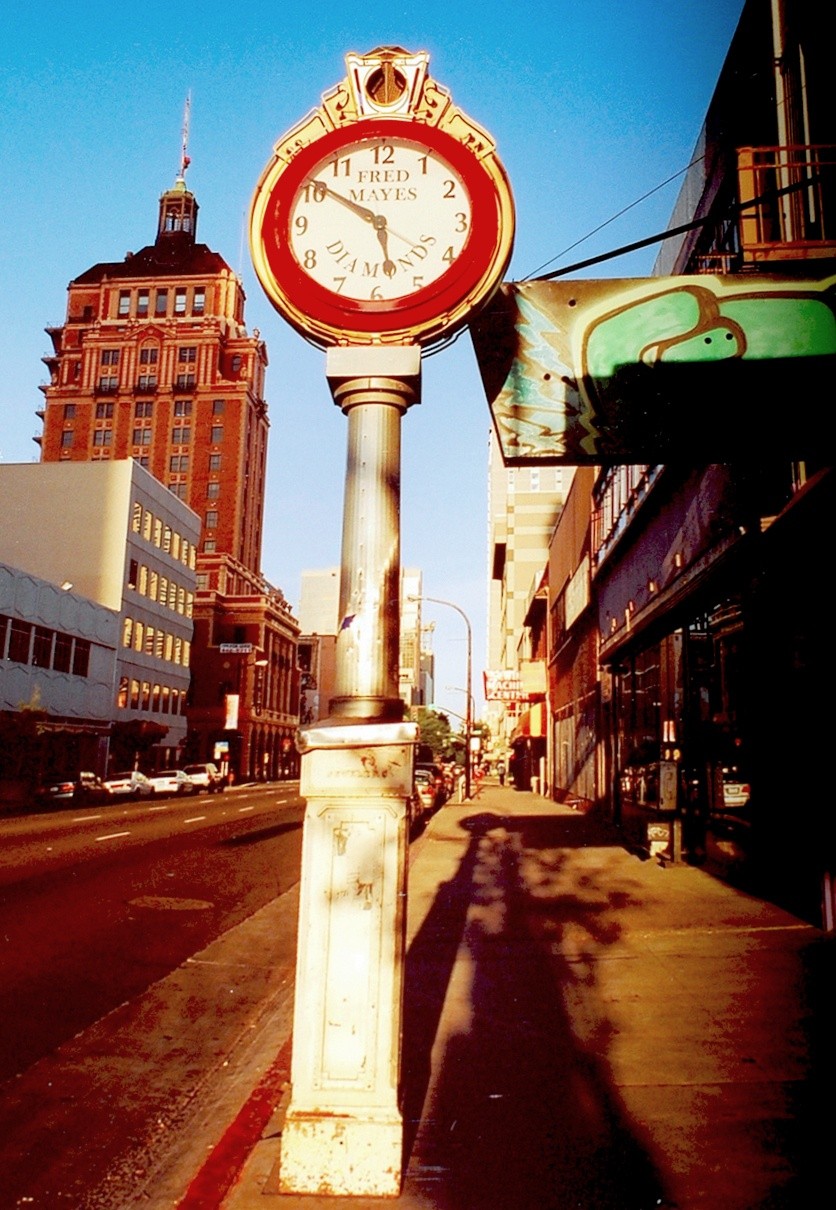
499;765;505;787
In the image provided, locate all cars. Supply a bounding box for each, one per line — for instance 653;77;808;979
35;769;110;810
690;774;752;811
103;771;154;802
150;770;197;799
412;760;489;823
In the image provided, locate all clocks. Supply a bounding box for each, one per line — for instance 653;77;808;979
281;129;478;314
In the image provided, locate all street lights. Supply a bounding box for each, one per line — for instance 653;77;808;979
236;660;269;696
408;594;472;802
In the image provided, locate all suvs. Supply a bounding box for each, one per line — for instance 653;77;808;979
184;763;224;795
